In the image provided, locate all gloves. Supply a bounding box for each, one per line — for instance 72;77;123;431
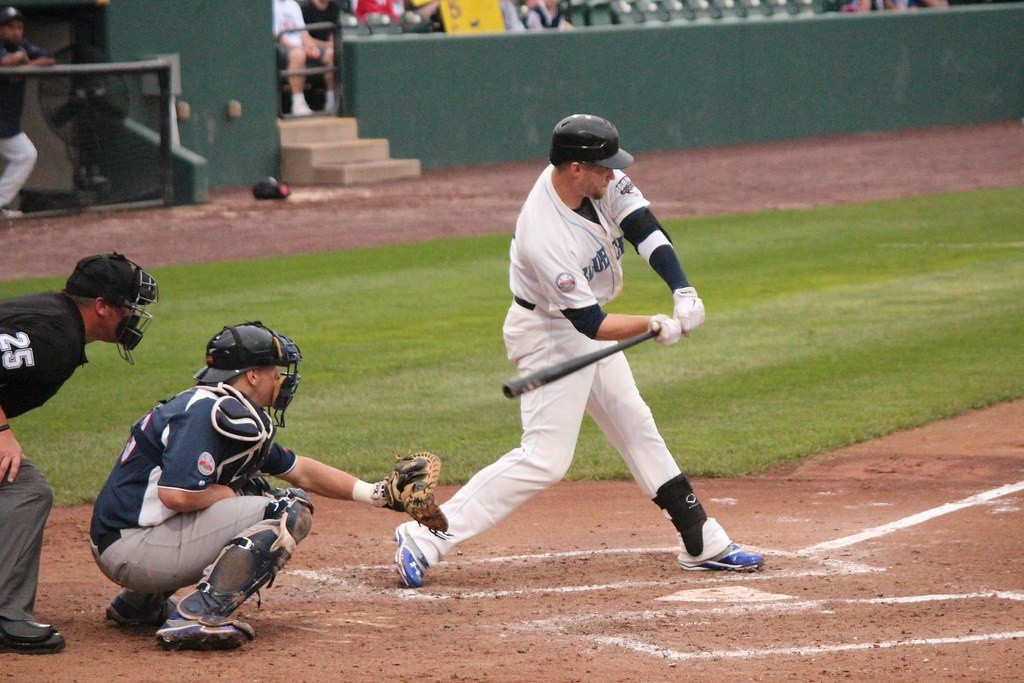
673;287;706;336
647;313;683;345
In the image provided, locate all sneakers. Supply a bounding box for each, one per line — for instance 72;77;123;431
105;593;179;629
393;522;431;588
677;544;767;575
155;608;250;652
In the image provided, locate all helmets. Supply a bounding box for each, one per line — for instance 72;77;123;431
548;112;636;170
192;321;303;383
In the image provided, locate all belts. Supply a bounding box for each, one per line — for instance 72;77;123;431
512;297;537;310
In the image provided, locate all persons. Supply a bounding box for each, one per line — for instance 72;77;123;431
840;0;952;13
501;0;573;32
0;252;160;652
395;115;764;587
0;8;55;219
90;320;448;651
275;0;444;117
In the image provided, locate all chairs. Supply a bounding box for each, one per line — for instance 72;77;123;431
339;0;824;36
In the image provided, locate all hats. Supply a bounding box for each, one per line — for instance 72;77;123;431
69;252;157;304
0;6;25;24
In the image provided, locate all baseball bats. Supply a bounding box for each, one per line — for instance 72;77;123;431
502;330;659;398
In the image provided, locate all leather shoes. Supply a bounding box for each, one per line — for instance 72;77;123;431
0;621;67;656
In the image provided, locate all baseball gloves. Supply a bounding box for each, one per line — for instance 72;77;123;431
382;452;448;532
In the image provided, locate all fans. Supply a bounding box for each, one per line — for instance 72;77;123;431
36;45;129;203
